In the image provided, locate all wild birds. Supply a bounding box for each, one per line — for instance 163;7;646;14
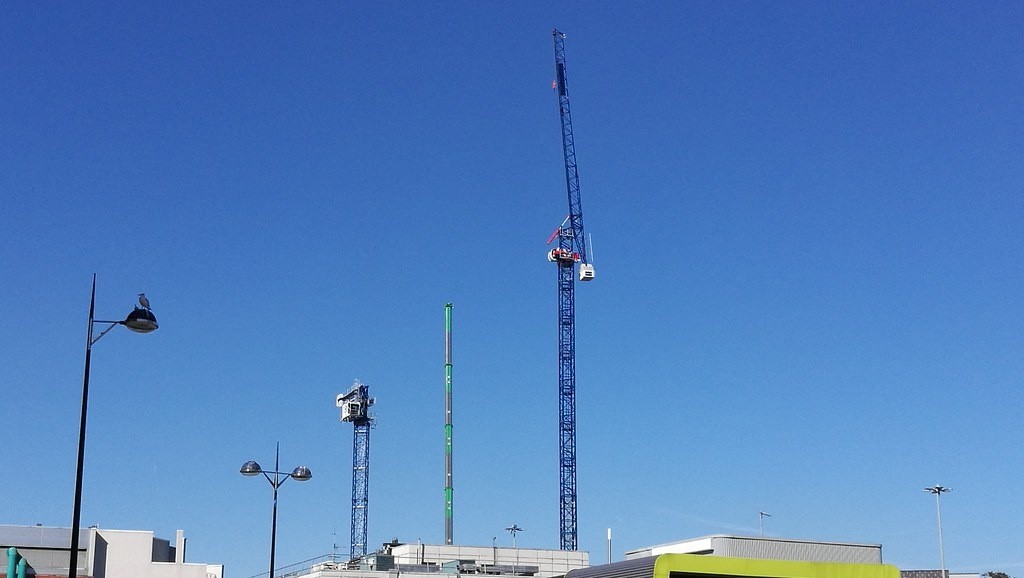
138;292;151;311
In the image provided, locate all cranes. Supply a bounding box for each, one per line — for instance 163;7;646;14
549;27;596;551
334;380;379;564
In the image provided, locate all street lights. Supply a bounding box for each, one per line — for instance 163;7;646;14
505;524;524;550
65;273;160;578
923;486;953;578
240;438;313;578
